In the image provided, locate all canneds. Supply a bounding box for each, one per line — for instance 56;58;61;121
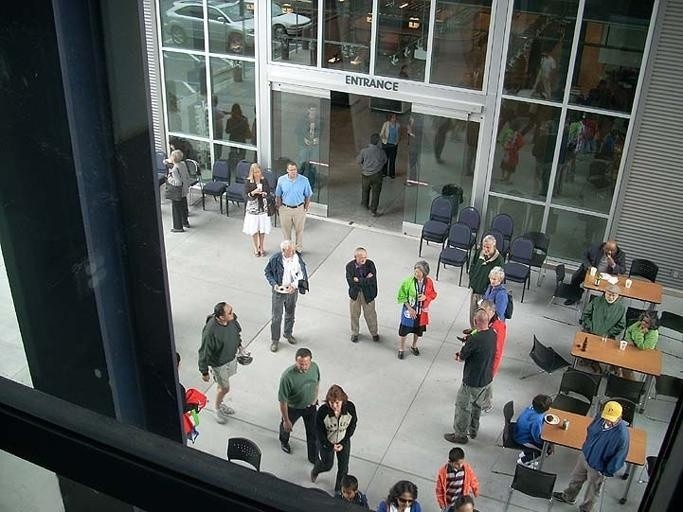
563;419;570;431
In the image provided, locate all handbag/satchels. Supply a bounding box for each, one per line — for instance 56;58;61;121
164;178;184;203
262;177;276;217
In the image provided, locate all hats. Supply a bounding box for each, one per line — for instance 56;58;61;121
599;399;624;423
603;283;622;295
236;352;254;366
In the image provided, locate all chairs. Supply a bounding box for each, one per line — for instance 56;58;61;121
636;456;659;485
435;221;472;288
506;464;558;512
621;257;659;310
651;311;683;360
620;306;660;341
457;205;480;269
489;213;514;263
154;134;325;228
489;400;525;477
467;228;507;291
441;182;464;224
225;437;263;473
502;235;536;303
543;263;585;326
519;334;572;398
418;194;455;259
524;231;550;288
554;367;604;418
600;371;647;429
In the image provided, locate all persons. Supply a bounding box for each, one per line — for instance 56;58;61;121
197;301;247;424
278;347;357;491
345;247;380;342
166;138;191;233
174;353;200;442
434;448;480;512
205;96;257;171
398;65;409;81
290;102;321;191
263;240;309;353
442;235;508;445
580;282;659;383
551;401;630;511
516;395;555;469
428;36;638;199
395;261;437;359
355;111;420;216
242;162;313;257
563;241;625;305
334;475;422;512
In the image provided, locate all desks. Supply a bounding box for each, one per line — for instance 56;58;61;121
570;331;663;415
539;407;649;504
577;272;665;325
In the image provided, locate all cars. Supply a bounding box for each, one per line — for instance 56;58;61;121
242;0;313;42
162;1;255;55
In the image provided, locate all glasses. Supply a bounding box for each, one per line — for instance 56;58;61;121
394;495;415;504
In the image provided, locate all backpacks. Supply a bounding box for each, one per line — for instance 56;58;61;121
493;288;514;320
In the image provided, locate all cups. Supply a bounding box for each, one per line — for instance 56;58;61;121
256;184;262;191
620;340;626;351
624;278;632;289
602;333;607;342
562;418;567;429
591;267;596;277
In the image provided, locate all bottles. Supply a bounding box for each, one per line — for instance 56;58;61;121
594;271;601;287
582;336;587;351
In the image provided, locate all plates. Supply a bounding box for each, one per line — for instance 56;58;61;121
276;286;290;294
544;413;559;425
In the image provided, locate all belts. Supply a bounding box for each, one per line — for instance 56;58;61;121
279;201;305;209
